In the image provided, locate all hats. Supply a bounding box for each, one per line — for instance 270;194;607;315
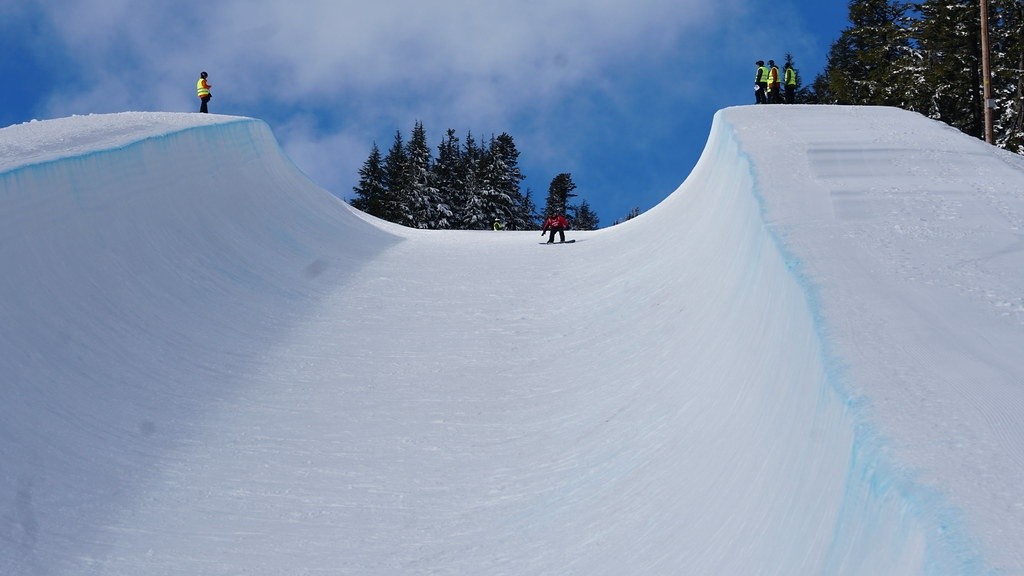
785;62;790;68
756;61;764;66
767;60;774;65
495;218;500;221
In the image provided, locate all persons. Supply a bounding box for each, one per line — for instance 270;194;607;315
784;63;796;104
766;60;784;104
196;71;213;114
541;210;568;244
493;218;504;231
753;61;767;104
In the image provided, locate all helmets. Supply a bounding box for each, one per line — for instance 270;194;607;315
201;71;207;77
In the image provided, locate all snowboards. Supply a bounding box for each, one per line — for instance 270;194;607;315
539;240;575;244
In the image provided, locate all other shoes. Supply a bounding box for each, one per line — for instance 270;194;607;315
561;239;565;243
547;240;554;244
